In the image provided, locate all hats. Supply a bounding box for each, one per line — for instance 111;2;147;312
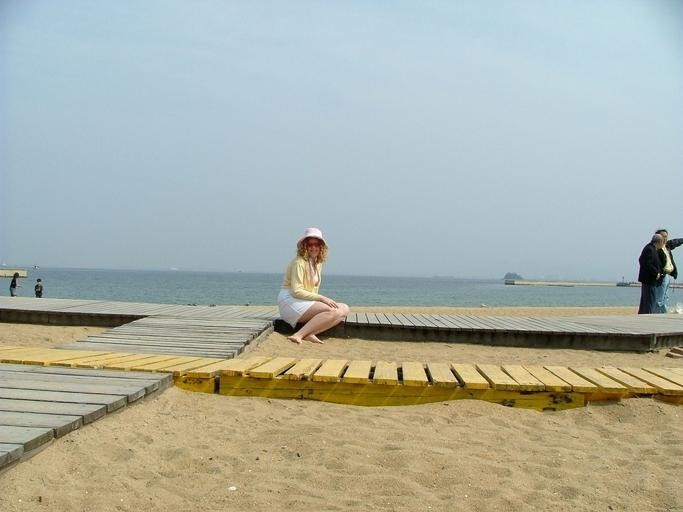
300;228;325;246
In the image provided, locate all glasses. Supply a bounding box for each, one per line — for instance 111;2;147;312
308;243;320;247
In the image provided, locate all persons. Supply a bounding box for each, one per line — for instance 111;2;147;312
275;226;350;346
655;230;683;313
637;232;667;315
9;272;21;296
34;278;43;298
